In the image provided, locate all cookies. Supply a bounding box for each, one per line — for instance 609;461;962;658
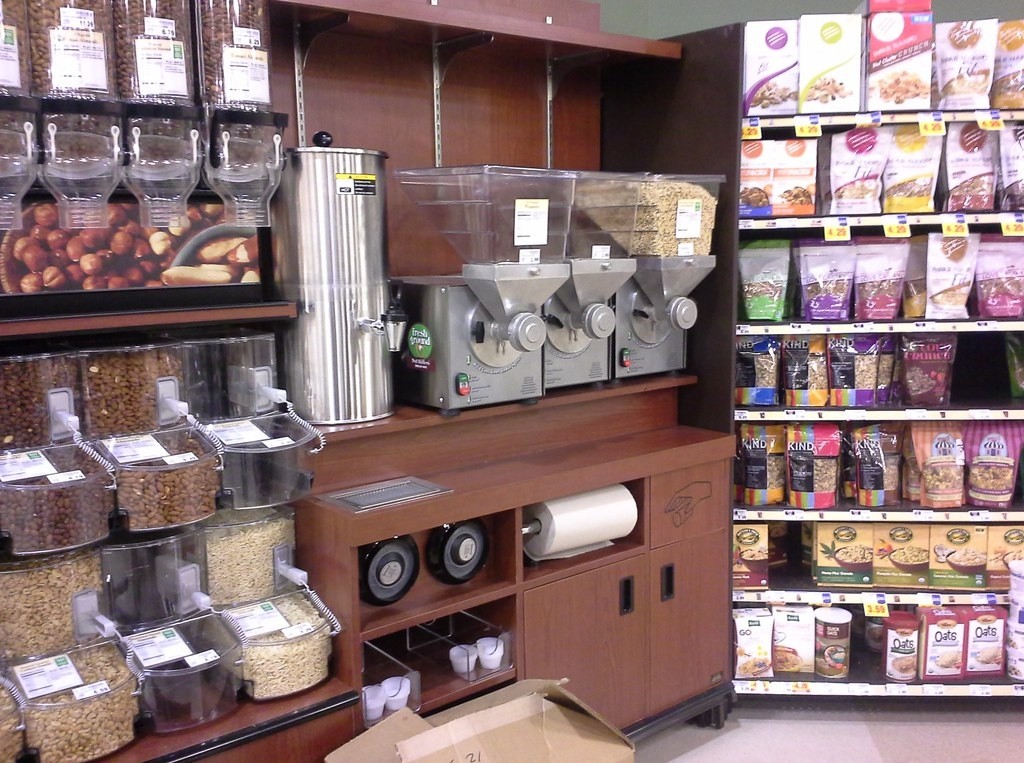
741;188;769;207
782;187;812;205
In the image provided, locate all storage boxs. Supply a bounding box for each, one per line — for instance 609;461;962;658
986;526;1024;591
732;524;769;590
817;522;874;588
873;523;930;589
739;140;772;218
771;605;816;672
744;20;798;116
766;521;788;567
797;14;862;114
323;679;636;763
851;0;932;18
732;607;775;679
801;521;813;565
957;606;1008;678
929;525;988;592
913;606;968;682
865;11;933;112
772;139;818;218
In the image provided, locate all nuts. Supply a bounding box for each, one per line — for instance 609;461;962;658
0;350;331;763
13;204;225;295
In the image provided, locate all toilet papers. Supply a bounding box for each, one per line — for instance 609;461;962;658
523;482;639;561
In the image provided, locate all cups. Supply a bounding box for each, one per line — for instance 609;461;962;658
362;677;410;721
449;637;504;673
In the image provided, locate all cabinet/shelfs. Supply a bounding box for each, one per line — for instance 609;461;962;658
0;299;361;763
600;21;1024;696
524;531;730;730
